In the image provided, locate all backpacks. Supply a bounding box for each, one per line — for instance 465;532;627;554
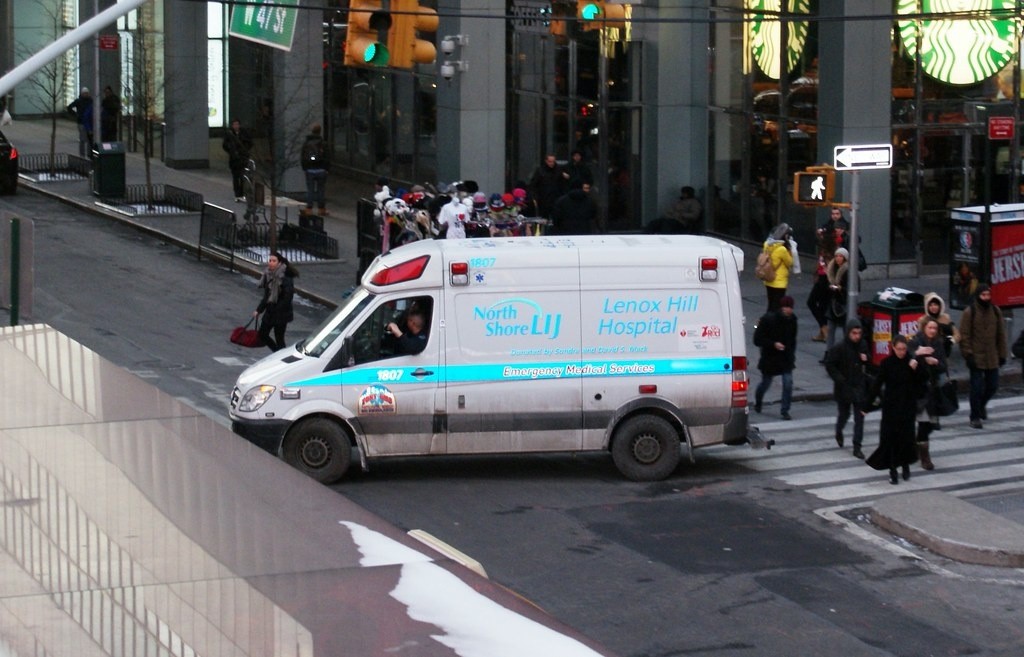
934;319;955;357
755;244;784;282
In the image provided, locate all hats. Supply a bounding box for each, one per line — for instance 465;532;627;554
835;248;849;261
781;296;794;309
975;284;992;295
376;176;528;224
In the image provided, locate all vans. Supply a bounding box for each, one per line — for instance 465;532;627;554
231;235;751;484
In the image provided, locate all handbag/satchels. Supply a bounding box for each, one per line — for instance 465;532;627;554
230;314;266;347
925;353;959;416
848;357;883;395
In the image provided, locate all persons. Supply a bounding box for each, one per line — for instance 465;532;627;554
252;250;300;354
377;310;428;357
752;296;799;420
67;87;95;161
300;124;333;216
385;299;424;339
0;62;15;120
641;186;704;235
762;206;1024;485
437;196;471;239
222;117;255;203
528;149;631;235
100;84;121;143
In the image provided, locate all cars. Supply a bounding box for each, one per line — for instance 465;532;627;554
0;130;18;193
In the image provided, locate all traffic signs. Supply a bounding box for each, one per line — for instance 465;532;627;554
834;144;893;171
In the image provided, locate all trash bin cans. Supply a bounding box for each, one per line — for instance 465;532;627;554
870;287;926;369
88;141;124;196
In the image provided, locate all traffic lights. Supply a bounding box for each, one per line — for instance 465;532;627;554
575;0;625;32
793;171;833;204
344;0;392;69
386;0;437;71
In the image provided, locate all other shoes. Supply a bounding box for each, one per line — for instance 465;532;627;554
234;197;247;203
297;208;313;215
979;405;987;419
969;418;983;429
853;445;866;459
317;208;330;216
781;408;791;420
901;464;911;480
889;468;898;484
835;423;844;447
754;400;763;413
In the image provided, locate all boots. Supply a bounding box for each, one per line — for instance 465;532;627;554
916;441;934;470
812;326;830;342
